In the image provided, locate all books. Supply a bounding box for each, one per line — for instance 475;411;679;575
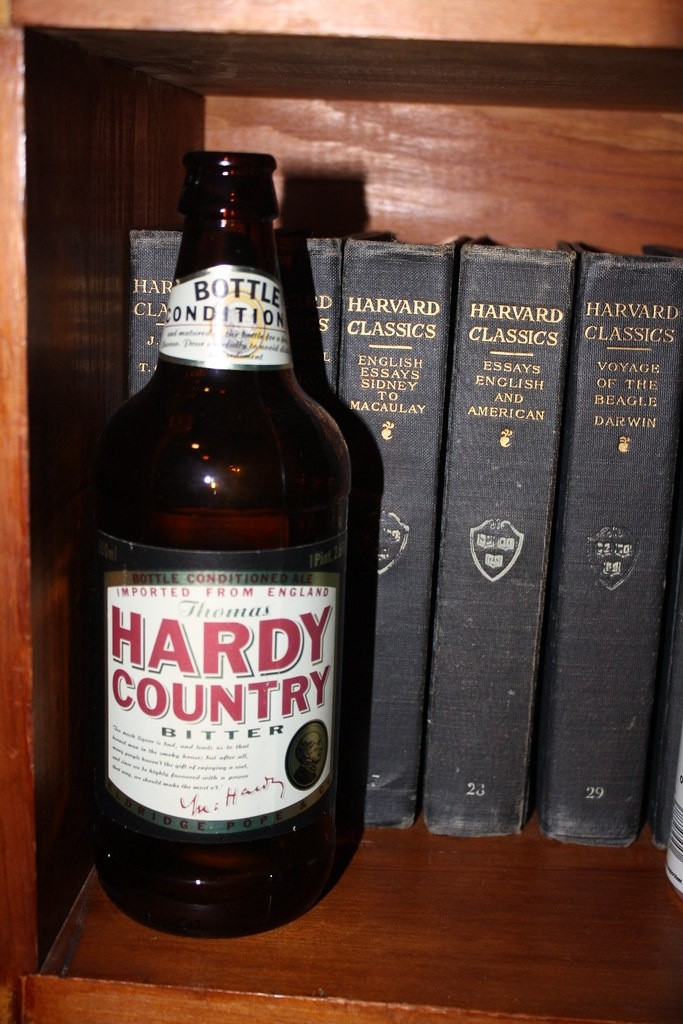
535;232;683;847
268;216;338;417
127;210;183;404
332;232;465;832
423;232;580;839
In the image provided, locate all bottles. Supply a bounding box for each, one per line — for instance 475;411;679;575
83;148;360;936
